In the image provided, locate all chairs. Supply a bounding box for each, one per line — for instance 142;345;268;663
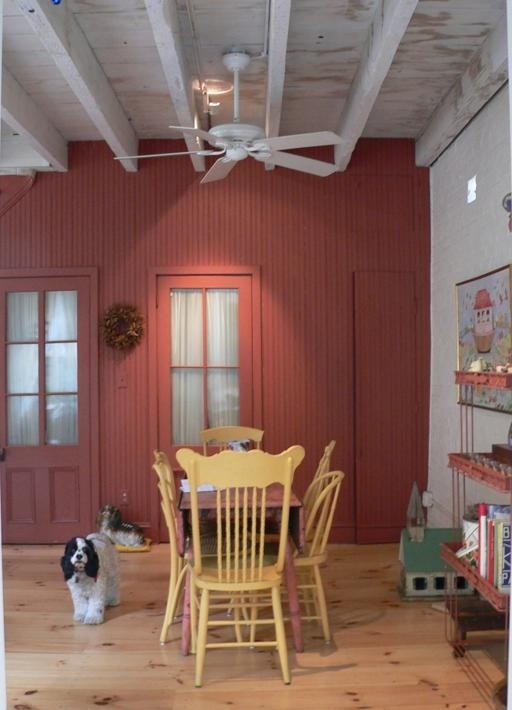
200;427;265;455
244;439;344;649
175;445;308;686
153;449;247;649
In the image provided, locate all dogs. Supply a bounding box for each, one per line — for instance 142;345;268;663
96;503;146;548
60;532;120;625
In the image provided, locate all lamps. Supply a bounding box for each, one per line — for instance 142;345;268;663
203;85;221;115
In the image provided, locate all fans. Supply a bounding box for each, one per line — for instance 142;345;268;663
114;53;344;184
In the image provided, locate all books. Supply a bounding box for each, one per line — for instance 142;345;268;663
466;499;511;597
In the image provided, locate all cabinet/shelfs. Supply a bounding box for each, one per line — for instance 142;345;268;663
441;370;512;710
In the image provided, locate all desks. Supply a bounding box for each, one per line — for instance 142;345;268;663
173;477;309;657
